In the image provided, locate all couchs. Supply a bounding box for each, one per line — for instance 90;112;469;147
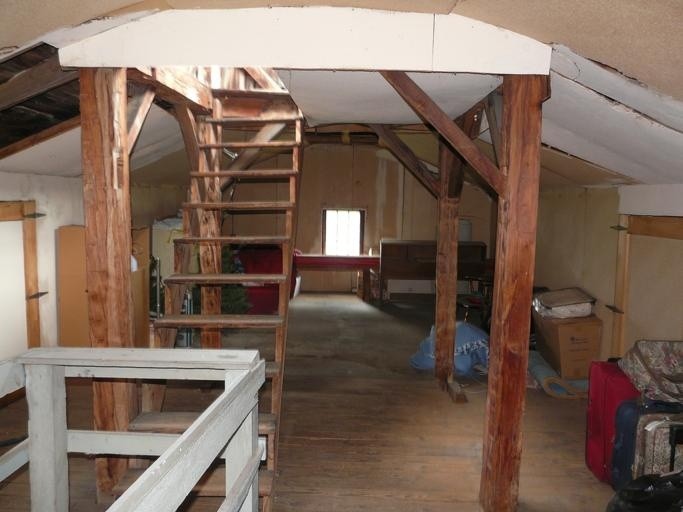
233;246;296;311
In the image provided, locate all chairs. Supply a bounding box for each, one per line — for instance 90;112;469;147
458;274;493;324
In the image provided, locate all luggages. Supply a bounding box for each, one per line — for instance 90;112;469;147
585;358;683;493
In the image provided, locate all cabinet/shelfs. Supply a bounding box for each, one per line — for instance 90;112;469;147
378;239;488;308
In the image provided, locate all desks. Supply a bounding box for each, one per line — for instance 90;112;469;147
296;253;379;302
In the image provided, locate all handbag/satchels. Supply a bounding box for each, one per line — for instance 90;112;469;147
618;339;682;402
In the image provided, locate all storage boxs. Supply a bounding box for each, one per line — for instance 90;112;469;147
531;309;603;379
